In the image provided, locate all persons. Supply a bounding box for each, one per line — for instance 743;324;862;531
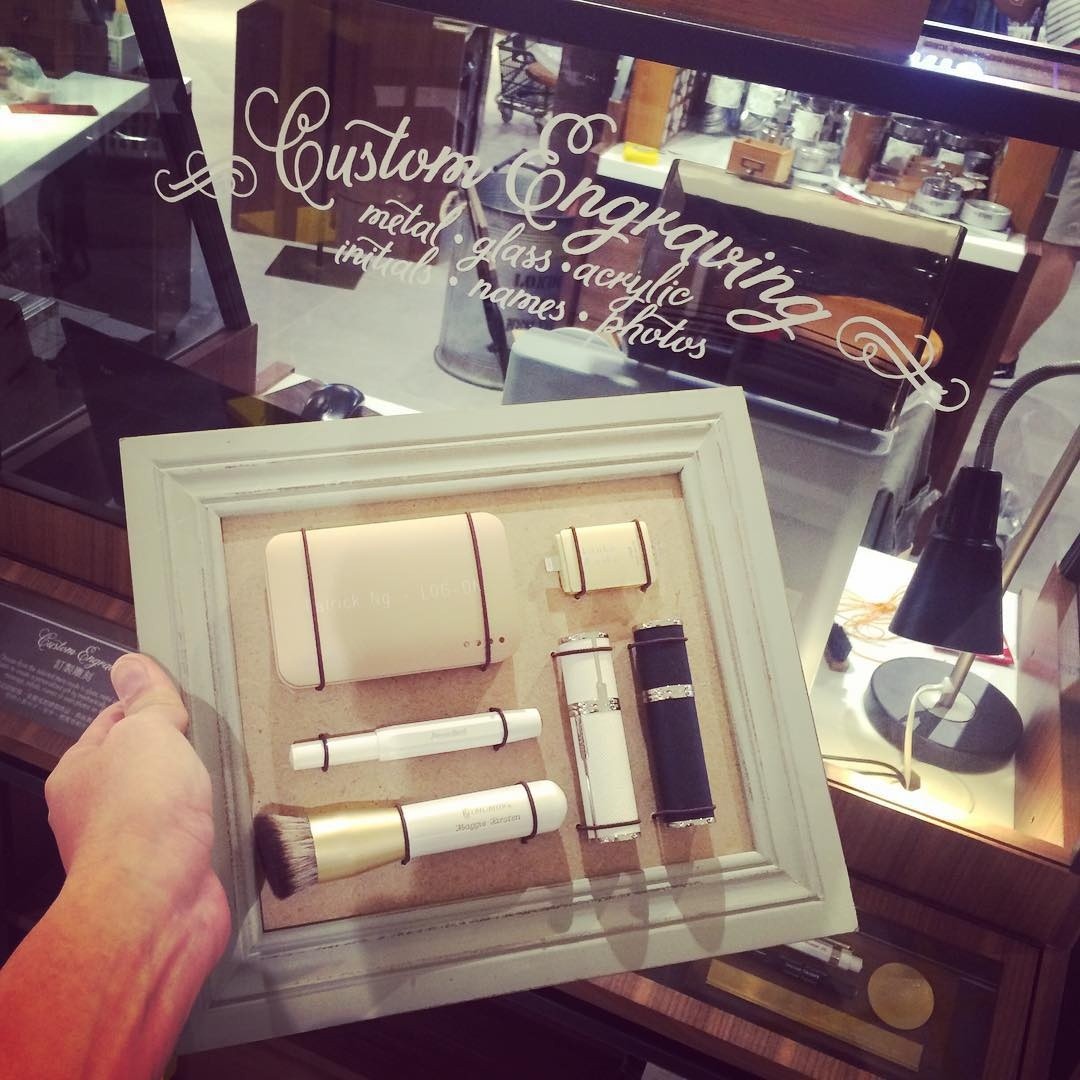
988;0;1080;387
0;653;233;1080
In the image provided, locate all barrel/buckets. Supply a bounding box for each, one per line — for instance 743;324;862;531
431;173;574;389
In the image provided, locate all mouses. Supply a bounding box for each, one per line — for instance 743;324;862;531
300;383;365;424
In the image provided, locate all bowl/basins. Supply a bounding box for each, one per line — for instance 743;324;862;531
793;147;832;174
959;198;1012;231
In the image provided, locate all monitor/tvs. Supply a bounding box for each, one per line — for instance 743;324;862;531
57;316;310;506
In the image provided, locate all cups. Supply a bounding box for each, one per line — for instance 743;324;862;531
964;147;995;175
892;116;938;145
837;107;890;185
938;128;974;154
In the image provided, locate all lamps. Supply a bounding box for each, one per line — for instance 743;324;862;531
864;362;1080;774
